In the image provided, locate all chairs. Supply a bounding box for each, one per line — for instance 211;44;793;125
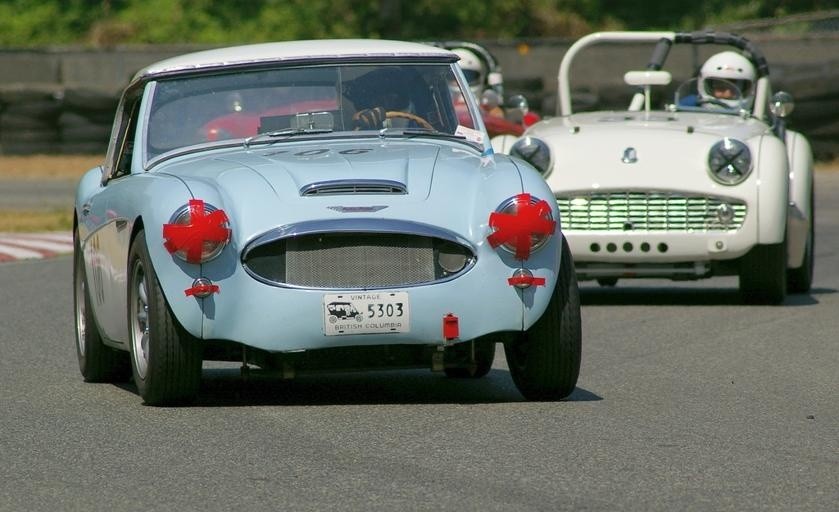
589;85;664;113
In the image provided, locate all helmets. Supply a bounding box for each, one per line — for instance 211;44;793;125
698;51;755;112
449;48;486;95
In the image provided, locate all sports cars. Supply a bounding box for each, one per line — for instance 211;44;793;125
417;40;543;138
508;28;817;305
65;36;585;409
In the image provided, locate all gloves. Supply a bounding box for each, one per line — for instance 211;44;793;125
352;105;386;128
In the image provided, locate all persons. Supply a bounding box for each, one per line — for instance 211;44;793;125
346;68;418;133
444;47;486;104
676;51;758;115
